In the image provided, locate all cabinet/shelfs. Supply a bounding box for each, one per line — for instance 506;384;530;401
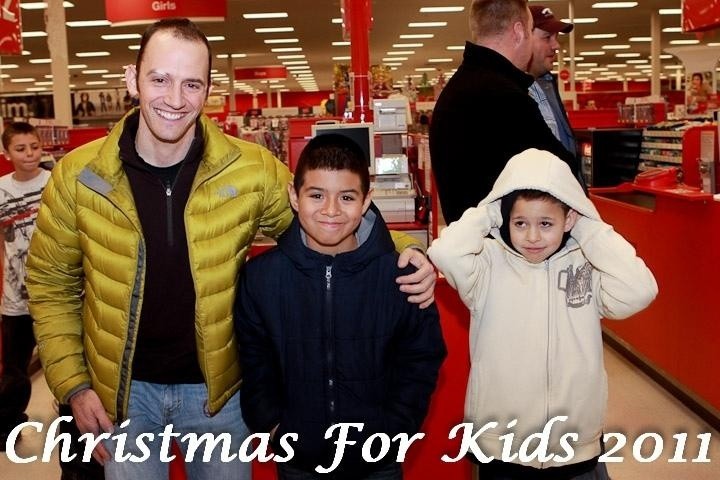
637;120;706;176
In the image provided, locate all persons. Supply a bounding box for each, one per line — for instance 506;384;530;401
114;87;122;112
0;119;55;452
99;91;107;113
425;148;661;480
525;6;591;203
24;19;440;480
229;132;449;480
427;2;575;230
124;89;132;111
77;92;95;116
106;92;113;113
687;73;712;96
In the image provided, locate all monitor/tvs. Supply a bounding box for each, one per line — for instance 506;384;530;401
311;121;376;177
315;119;338;125
373;98;408;131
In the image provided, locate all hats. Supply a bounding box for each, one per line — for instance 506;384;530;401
525;4;575;36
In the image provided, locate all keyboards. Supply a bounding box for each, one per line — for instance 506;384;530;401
369;188;417;197
